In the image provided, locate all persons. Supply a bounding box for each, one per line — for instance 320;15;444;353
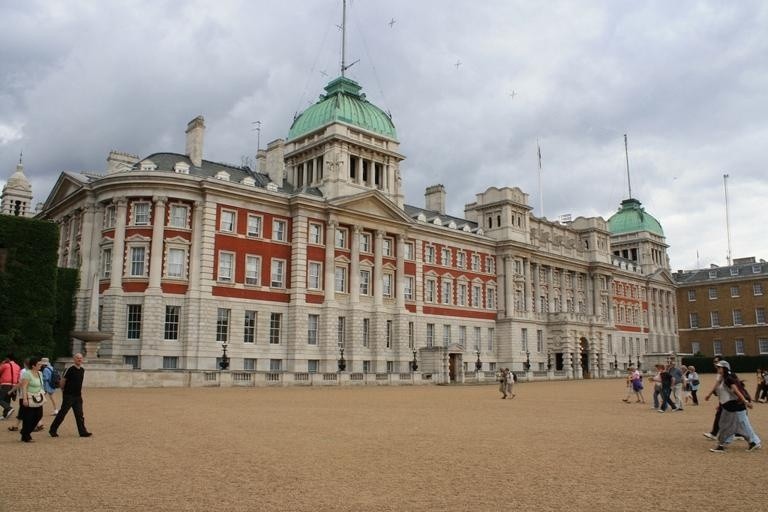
498;367;507;399
505;368;515;399
620;354;768;453
0;355;62;443
49;352;94;438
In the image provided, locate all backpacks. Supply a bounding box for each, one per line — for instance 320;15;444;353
44;367;61;388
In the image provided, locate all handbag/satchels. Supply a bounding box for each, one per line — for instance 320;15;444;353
26;392;46;408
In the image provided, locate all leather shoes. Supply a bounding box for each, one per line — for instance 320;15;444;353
710;449;724;453
744;444;759;452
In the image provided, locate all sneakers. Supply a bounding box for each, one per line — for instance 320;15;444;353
732;437;743;441
702;433;716;440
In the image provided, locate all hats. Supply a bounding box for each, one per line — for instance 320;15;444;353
716;361;729;370
40;358;50;365
